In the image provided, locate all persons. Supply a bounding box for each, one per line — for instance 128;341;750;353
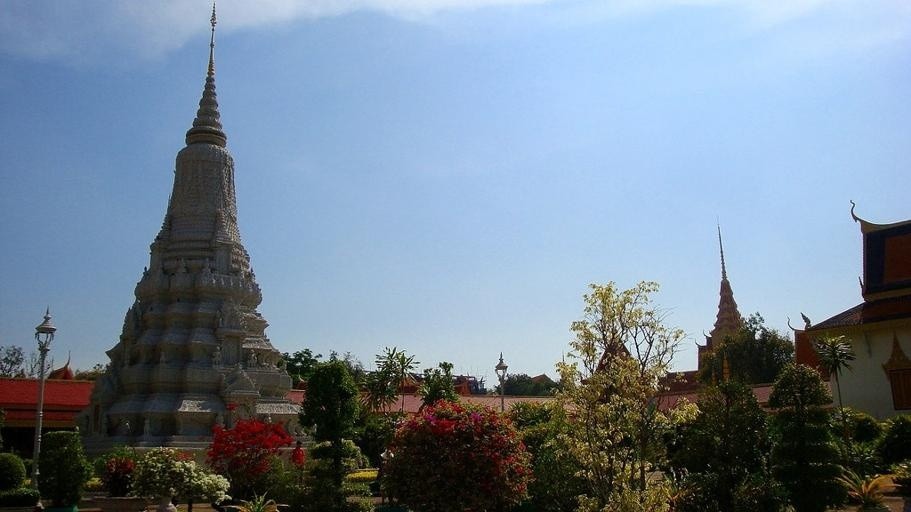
291;442;305;486
669;466;687;483
380;450;394;505
359;460;363;469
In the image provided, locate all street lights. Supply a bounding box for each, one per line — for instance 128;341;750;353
493;350;510;417
29;304;58;509
